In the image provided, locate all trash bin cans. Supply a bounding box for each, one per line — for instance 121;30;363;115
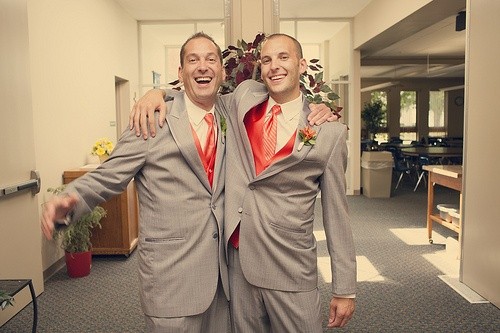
362;151;394;197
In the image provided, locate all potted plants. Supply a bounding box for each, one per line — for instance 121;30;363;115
47;186;107;277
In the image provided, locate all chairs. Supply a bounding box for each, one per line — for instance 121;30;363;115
380;137;451;192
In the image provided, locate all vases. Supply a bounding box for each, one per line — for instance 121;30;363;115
100;153;108;162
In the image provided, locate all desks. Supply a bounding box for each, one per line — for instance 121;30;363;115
422;165;462;244
0;279;37;333
400;147;462;165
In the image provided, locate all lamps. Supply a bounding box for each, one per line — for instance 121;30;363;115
360;63;399;92
427;52;465;76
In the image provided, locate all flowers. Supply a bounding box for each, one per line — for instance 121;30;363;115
297;125;317;151
92;137;114;156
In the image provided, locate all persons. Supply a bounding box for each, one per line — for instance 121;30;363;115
41;36;337;333
128;34;364;333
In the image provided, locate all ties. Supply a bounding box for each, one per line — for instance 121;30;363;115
262;105;282;163
203;112;216;165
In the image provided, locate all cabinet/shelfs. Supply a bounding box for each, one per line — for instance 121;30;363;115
63;167;138;258
